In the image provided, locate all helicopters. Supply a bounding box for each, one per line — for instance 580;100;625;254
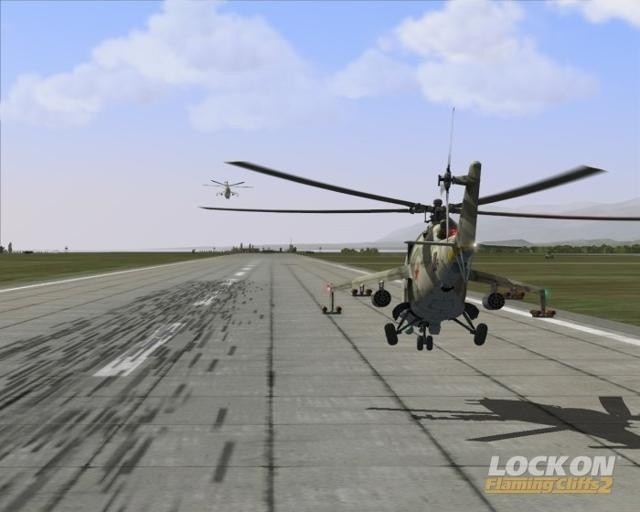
202;177;251;201
199;104;640;349
